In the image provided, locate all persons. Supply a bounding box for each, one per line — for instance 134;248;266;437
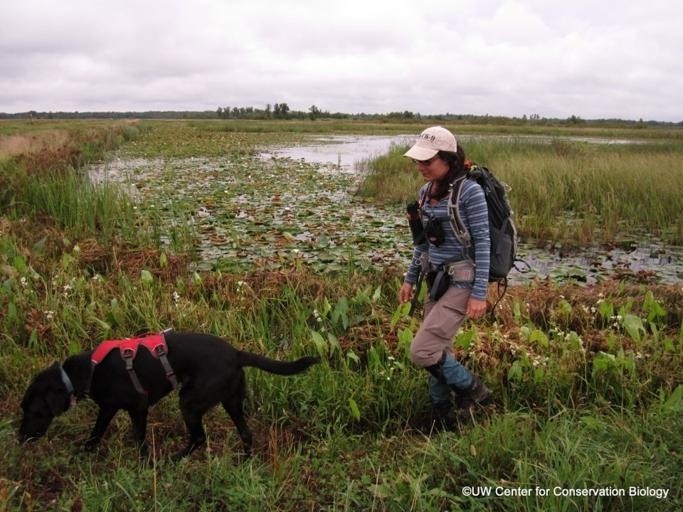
396;123;495;435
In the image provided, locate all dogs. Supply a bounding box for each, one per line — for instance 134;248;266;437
18;329;321;464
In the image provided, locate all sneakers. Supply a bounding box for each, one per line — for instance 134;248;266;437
414;400;452;425
456;377;493;418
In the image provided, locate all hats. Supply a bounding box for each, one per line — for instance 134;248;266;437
402;126;457;161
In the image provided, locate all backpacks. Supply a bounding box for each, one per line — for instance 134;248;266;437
449;160;517;281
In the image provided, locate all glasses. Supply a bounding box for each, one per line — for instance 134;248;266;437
412;156;438;166
